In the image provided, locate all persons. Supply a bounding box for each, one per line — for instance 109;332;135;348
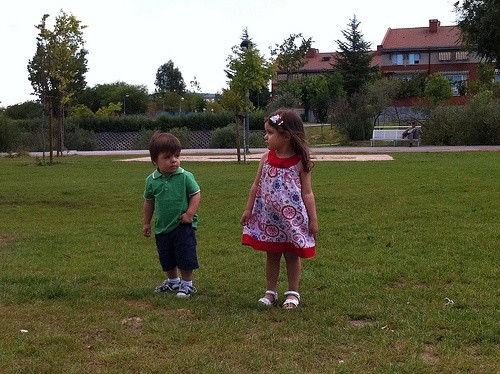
143;132;201;298
402;122;423;147
240;108;319;309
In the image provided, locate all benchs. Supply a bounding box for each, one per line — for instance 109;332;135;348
371;126;421;147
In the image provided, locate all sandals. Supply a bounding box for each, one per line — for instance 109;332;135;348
258;290;279;308
282;291;301;310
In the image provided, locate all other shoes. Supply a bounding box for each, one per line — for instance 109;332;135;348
176;283;197;299
154;280;182;294
